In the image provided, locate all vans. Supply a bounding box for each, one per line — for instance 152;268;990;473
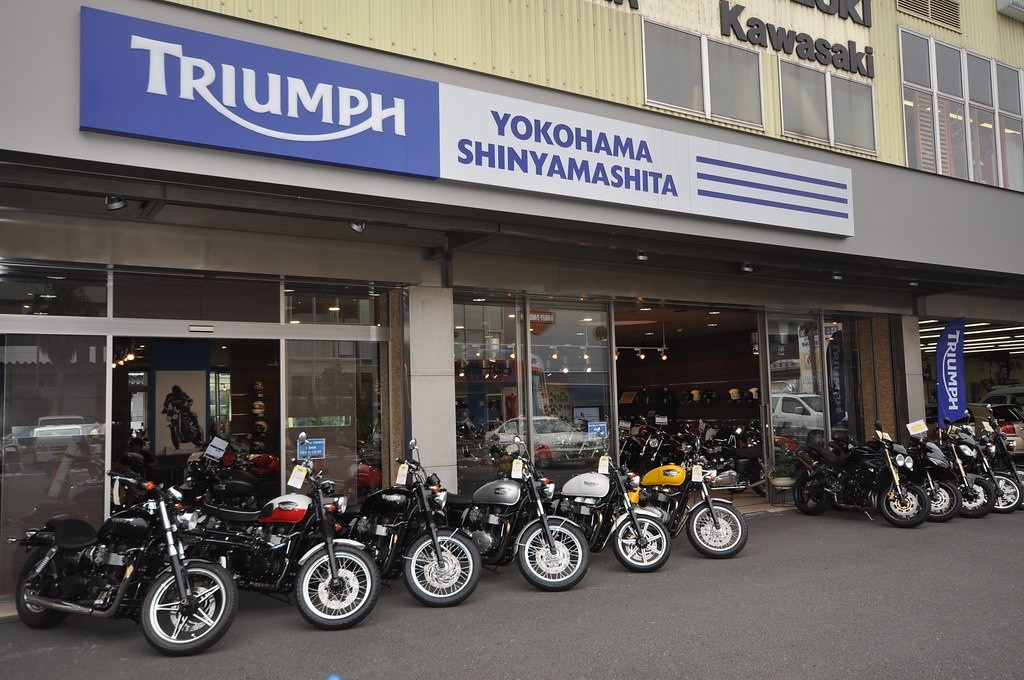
33;414;89;462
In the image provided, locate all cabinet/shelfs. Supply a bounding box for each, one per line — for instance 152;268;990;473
214;364;281;452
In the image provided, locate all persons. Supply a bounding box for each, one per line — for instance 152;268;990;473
161;385;203;437
1;429;27;453
128;439;164;484
91;424;105;441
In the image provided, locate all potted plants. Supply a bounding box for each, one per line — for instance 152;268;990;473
765;435;803;487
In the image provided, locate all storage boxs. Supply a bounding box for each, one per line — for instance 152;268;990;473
285;298;372;507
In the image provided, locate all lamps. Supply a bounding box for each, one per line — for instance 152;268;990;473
453;345;668;380
113;343;147;368
832;271;845;281
105;193;128;211
742;262;755;272
349;218;367;234
907;281;920;287
635;250;649;261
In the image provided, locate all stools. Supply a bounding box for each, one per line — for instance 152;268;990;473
772;486;795;508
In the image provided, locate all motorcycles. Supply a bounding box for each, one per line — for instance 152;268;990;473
6;428;671;656
623;440;749;558
791;405;1024;529
611;413;800;498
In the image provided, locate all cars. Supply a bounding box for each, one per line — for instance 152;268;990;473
926;382;1024;457
484;416;605;468
771;392;824;429
